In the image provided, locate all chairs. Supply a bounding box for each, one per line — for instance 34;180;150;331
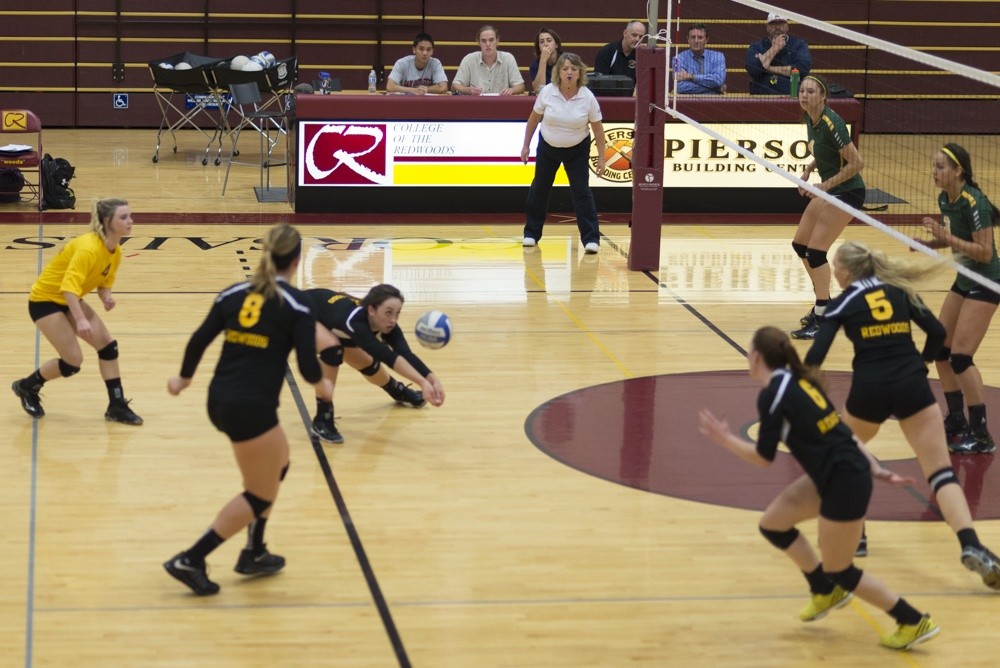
0;108;43;211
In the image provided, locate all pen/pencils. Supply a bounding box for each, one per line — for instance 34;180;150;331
470;82;477;88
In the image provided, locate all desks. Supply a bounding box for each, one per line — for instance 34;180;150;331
290;91;865;216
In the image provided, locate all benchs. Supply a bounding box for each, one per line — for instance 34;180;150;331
1;0;1000;135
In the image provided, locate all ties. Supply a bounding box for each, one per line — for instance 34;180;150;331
695;56;704;74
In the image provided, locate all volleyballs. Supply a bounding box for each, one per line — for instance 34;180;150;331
415;310;453;350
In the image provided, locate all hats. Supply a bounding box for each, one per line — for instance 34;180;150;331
766;13;789;24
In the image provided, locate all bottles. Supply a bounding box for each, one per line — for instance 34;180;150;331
319;72;331;95
790;68;800;97
368;70;376;93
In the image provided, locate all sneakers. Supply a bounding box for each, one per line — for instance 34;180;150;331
855;534;868;557
877;612;940;650
12;378;46;418
311;415;344;443
798;306;815;322
790;317;820;340
947;426;996;453
799;584;853;623
104;397;143;425
961;543;1000;590
164;551;221;594
233;542;287;574
943;412;967;431
390;382;427;408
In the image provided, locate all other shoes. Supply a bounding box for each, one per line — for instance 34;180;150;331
523;245;536;254
585;242;599;253
523;237;536;247
584;253;599;264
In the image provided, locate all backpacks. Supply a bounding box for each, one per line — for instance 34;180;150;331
0;168;37;203
41;153;76;210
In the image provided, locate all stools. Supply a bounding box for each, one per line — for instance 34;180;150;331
222;81;291;201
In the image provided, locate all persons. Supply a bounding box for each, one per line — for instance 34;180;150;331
671;24;727;96
909;144;1000;456
804;241;1000;591
519;51;608;253
450;25;526;96
593;20;646;87
527;27;563;95
791;74;868;340
698;324;942;650
387;34;449;94
302;283;445;442
162;226;330;597
13;199;144;426
743;11;813;96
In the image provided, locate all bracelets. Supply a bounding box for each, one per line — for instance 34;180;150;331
598;157;605;160
